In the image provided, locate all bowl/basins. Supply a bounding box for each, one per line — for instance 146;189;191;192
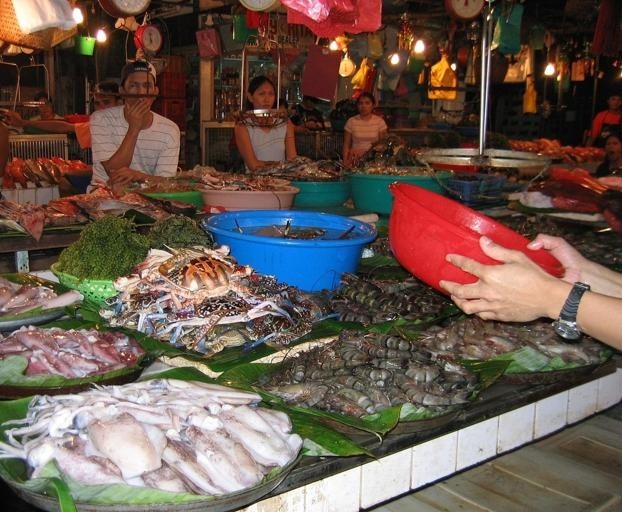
141;191;204;212
194;175;301;212
289;180;352;208
200;209;379;294
385;180;567;304
342;168;457;216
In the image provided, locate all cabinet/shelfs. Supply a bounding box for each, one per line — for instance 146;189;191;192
196;12;492;178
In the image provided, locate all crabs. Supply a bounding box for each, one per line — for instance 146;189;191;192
98;245;336;357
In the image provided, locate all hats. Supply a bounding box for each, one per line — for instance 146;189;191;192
120;59;156;86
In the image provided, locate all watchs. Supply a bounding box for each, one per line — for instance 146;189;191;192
550;281;592;342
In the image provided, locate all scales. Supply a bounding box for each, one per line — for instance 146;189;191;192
416;0;551;209
92;0;172;96
0;52;51;109
232;0;297;129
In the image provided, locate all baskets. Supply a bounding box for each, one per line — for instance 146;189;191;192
0;0;76;55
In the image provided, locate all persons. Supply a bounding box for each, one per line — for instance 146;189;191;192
234;75;298;176
584;87;621;147
24;90;67;133
0;79;126;149
588;131;622;179
342;91;389;164
437;230;622;354
84;57;181;195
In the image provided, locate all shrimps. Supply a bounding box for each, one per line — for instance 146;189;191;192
257;316;605;424
329;269;450;327
